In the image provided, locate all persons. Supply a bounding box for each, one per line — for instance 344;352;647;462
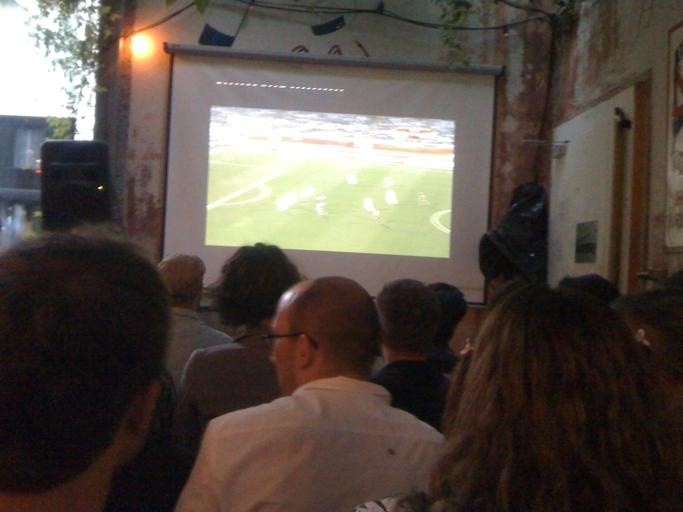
0;224;682;511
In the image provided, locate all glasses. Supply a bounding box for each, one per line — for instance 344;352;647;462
260;330;318;352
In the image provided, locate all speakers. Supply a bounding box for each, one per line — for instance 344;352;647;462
40;141;111;230
